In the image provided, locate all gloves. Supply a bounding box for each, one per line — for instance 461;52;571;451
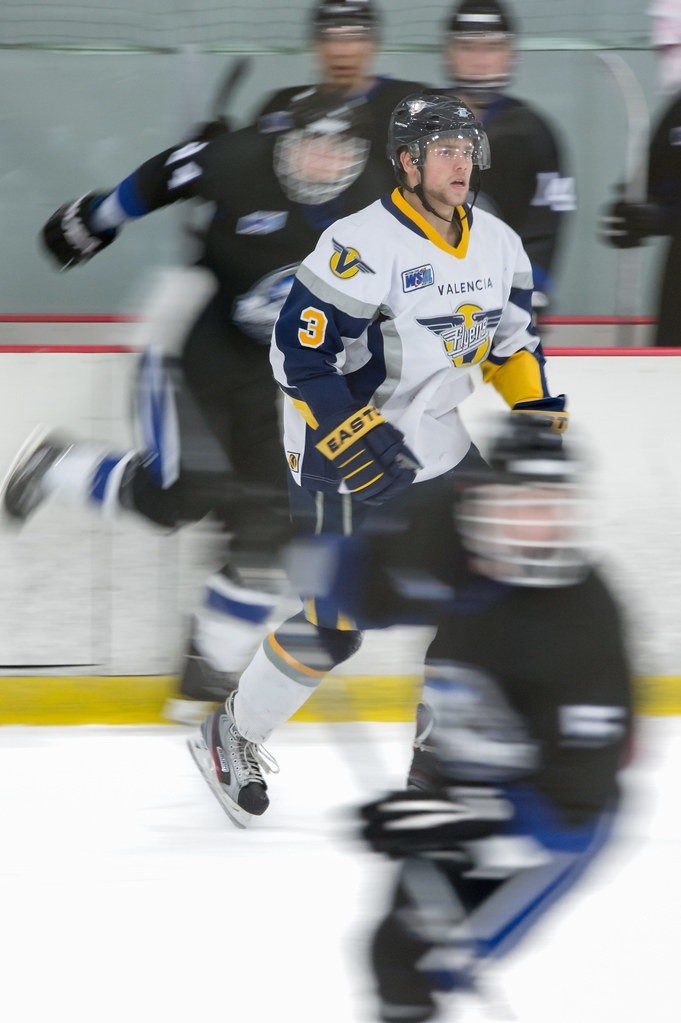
598;201;657;250
312;400;423;508
42;190;118;269
358;787;514;858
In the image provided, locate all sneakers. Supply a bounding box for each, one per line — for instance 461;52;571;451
185;690;280;829
2;423;68;530
163;645;241;724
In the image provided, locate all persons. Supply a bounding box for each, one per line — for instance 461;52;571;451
5;0;681;1023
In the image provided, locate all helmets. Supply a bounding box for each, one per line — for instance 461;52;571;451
387;93;483;174
267;77;376;202
447;0;510;41
449;421;614;585
312;0;379;33
